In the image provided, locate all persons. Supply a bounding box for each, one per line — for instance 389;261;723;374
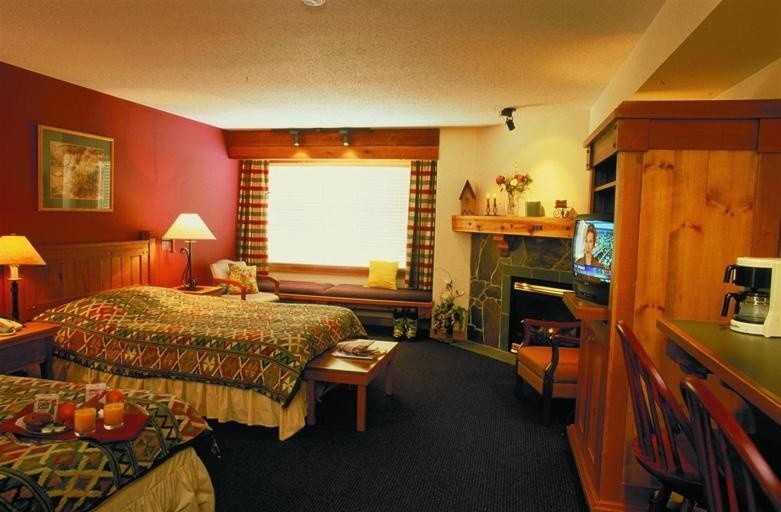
575;224;604;270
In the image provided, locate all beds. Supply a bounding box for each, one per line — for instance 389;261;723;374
5;231;370;441
0;370;223;511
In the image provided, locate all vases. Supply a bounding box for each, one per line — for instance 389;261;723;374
503;188;522;216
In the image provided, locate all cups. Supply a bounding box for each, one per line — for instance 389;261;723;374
526;200;540;217
74;408;96;437
103;403;124;430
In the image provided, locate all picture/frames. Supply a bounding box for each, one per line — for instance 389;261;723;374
36;122;116;213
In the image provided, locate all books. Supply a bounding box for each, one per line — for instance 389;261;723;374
332;350;374;360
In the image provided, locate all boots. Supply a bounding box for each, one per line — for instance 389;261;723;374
393;308;418;342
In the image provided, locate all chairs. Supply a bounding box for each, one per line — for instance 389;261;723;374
209;258;280;302
677;376;781;511
515;318;581;427
615;320;780;510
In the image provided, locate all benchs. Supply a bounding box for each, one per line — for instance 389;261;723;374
276;280;434;309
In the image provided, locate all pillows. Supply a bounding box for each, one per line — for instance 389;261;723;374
226;262;259;294
364;259;400;290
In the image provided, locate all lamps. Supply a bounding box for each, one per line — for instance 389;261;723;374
501;107;519;132
288;129;304;146
1;232;48;324
161;213;216;292
338;129;351;146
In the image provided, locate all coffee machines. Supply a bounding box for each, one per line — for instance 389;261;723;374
721;256;781;337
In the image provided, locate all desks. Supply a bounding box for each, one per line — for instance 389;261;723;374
656;316;781;423
302;337;399;431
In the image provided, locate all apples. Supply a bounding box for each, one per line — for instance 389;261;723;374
57;403;75;423
106;391;124;403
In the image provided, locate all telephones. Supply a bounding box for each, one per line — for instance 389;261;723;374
0;318;23;337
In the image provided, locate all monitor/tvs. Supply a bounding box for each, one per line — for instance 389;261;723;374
572;214;613;305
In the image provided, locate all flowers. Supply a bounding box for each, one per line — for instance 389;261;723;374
496;173;533;202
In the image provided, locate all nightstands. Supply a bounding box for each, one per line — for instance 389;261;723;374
1;319;61;380
170;285;223;297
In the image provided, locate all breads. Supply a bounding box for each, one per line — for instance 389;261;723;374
22;413;52;432
